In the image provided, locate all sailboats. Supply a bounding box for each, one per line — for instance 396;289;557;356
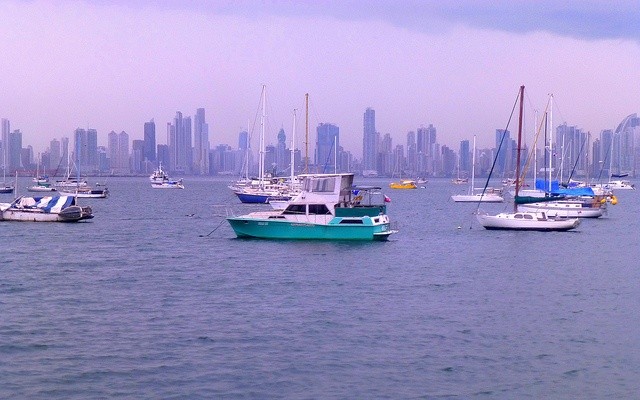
510;112;613;201
234;85;304;204
451;135;504;202
55;144;87;186
237;120;272;185
226;94;399;240
475;86;580;230
516;95;603;218
450;161;468;184
28;157;57;191
280;112;304;188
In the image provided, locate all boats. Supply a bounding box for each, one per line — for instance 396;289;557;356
1;195;94;222
60;188;109;198
1;185;13;192
389;181;417;190
607;180;635;190
148;161;185;190
31;175;47;182
500;177;529;186
415;179;427;184
